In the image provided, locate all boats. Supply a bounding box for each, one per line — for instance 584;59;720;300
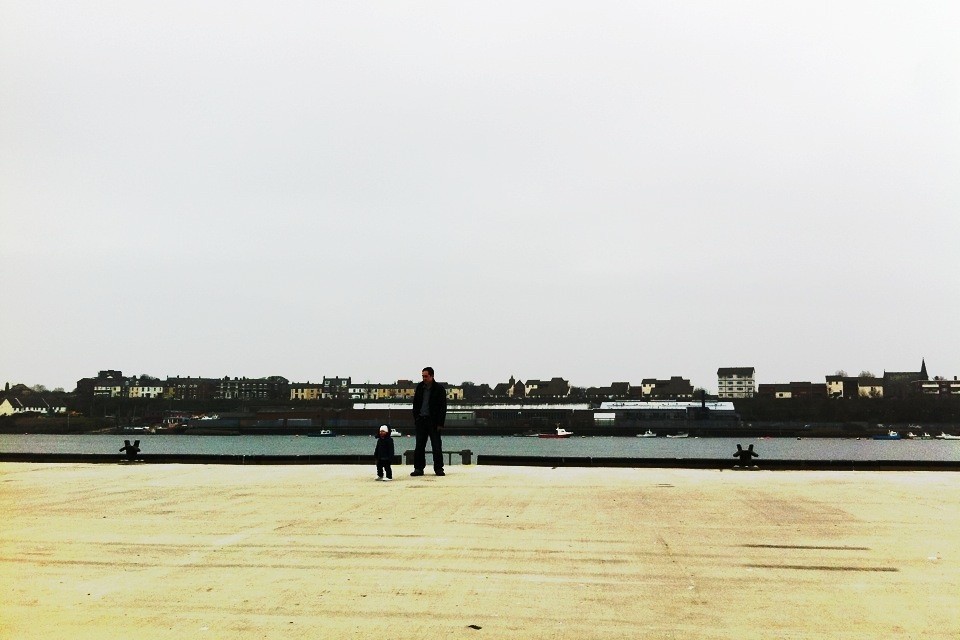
636;429;657;438
307;429;337;437
666;431;689;438
537;421;574;438
871;429;902;441
905;431;960;440
154;416;190;435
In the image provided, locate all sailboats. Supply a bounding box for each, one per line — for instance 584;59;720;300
387;407;402;437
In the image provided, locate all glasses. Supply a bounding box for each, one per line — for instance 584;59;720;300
422;374;429;378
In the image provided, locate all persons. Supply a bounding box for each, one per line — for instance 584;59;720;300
409;367;447;476
374;424;394;481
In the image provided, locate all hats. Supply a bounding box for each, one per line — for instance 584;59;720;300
380;425;388;432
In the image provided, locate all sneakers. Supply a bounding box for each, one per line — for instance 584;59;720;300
382;477;393;481
436;471;445;476
375;476;383;481
410;469;424;476
